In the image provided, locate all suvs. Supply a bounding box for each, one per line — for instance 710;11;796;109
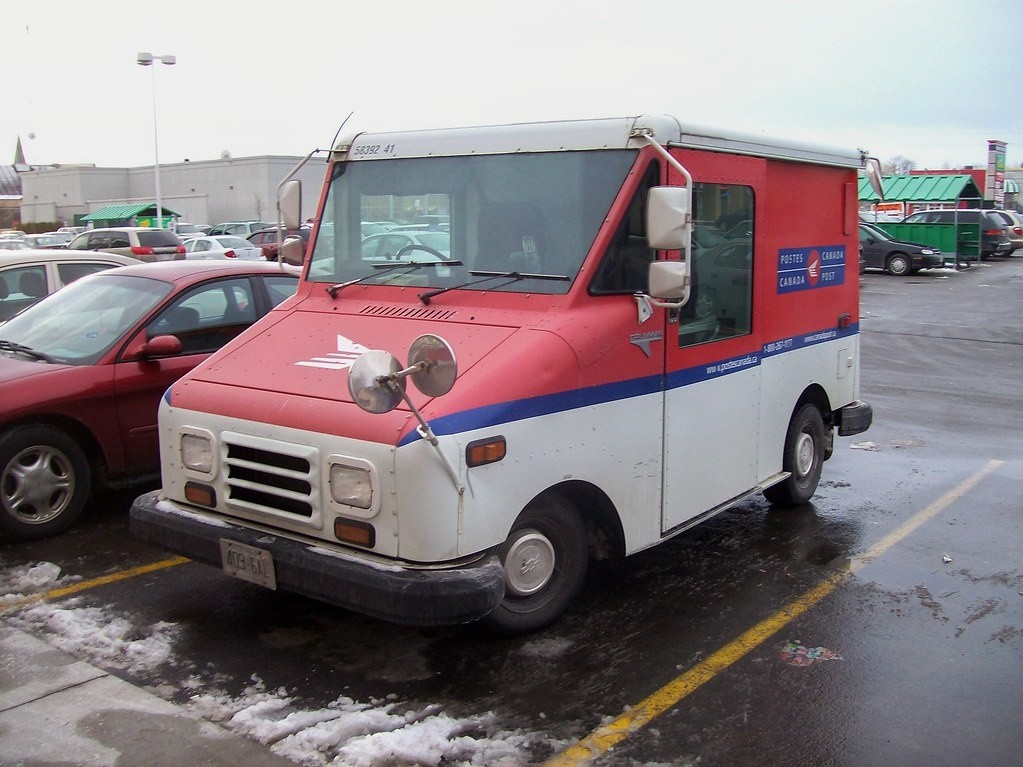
985;209;1022;256
65;227;181;266
900;209;1012;262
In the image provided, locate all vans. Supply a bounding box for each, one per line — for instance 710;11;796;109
127;108;873;640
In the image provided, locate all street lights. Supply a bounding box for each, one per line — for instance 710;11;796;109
138;52;179;231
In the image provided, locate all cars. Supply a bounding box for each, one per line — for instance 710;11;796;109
308;231;450;278
172;217;451;273
858;210;901;224
1;224;90;254
0;261;319;540
182;236;268;262
857;222;945;277
1;247;248;339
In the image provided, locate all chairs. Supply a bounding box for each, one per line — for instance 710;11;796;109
0;273;45;321
472;202;551;272
115;302;251;332
